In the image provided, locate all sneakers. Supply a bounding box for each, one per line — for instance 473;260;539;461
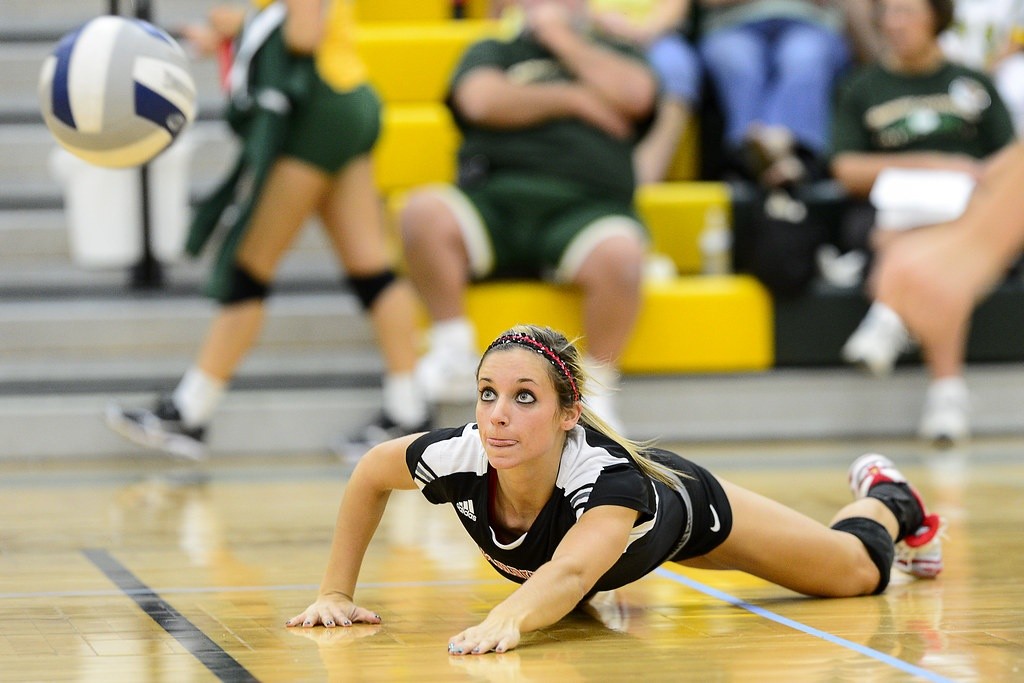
844;451;945;578
111;346;625;472
843;300;970;450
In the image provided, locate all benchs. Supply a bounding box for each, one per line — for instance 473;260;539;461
343;4;1024;365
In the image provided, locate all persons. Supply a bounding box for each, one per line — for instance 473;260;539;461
286;320;952;656
696;1;852;224
830;1;1022;441
109;1;441;457
576;3;696;188
401;1;666;450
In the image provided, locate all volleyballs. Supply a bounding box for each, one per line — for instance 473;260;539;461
36;14;200;169
